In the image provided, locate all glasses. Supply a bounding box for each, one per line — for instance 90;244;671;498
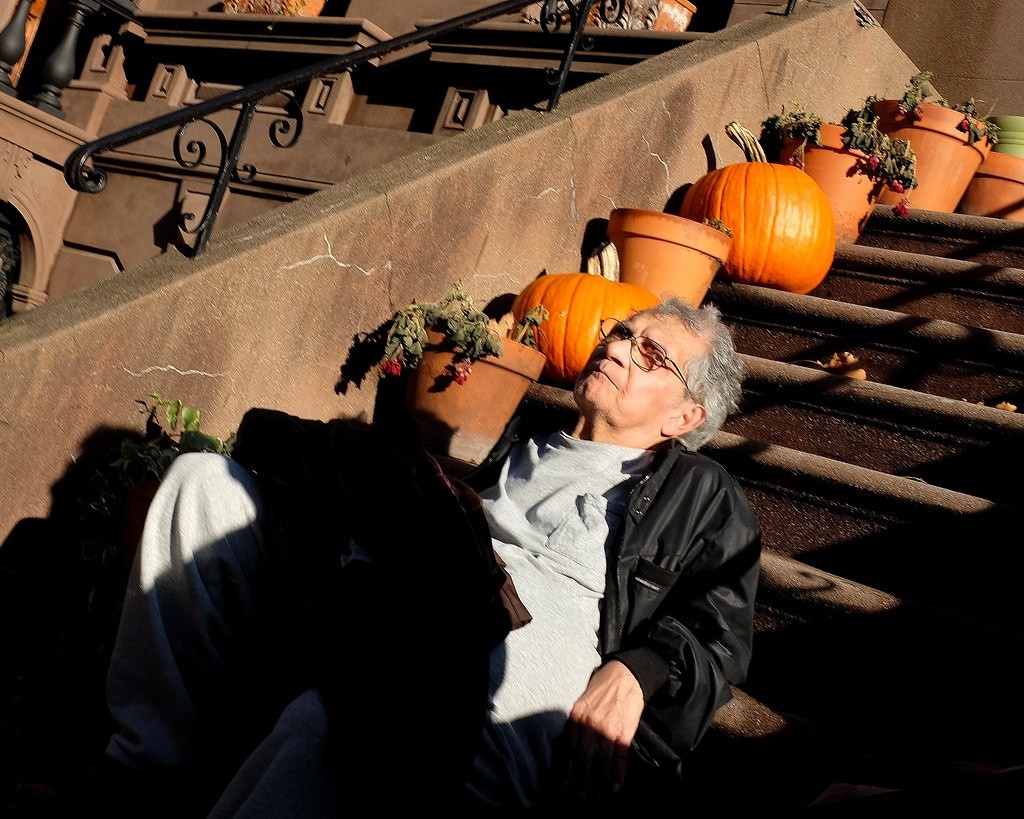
598;317;705;409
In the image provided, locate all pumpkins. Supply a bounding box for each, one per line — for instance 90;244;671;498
511;238;661;384
678;121;838;294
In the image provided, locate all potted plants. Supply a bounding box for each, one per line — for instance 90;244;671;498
605;208;732;308
76;395;219;553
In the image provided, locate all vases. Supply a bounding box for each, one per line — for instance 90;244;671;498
757;124;898;246
968;151;1024;220
386;329;546;467
868;102;994;211
988;116;1024;158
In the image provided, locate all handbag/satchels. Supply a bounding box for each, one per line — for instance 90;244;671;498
230;408;533;631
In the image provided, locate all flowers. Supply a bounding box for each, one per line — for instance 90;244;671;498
866;69;1001;142
752;108;916;214
378;278;551;385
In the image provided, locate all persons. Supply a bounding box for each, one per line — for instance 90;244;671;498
109;296;761;819
